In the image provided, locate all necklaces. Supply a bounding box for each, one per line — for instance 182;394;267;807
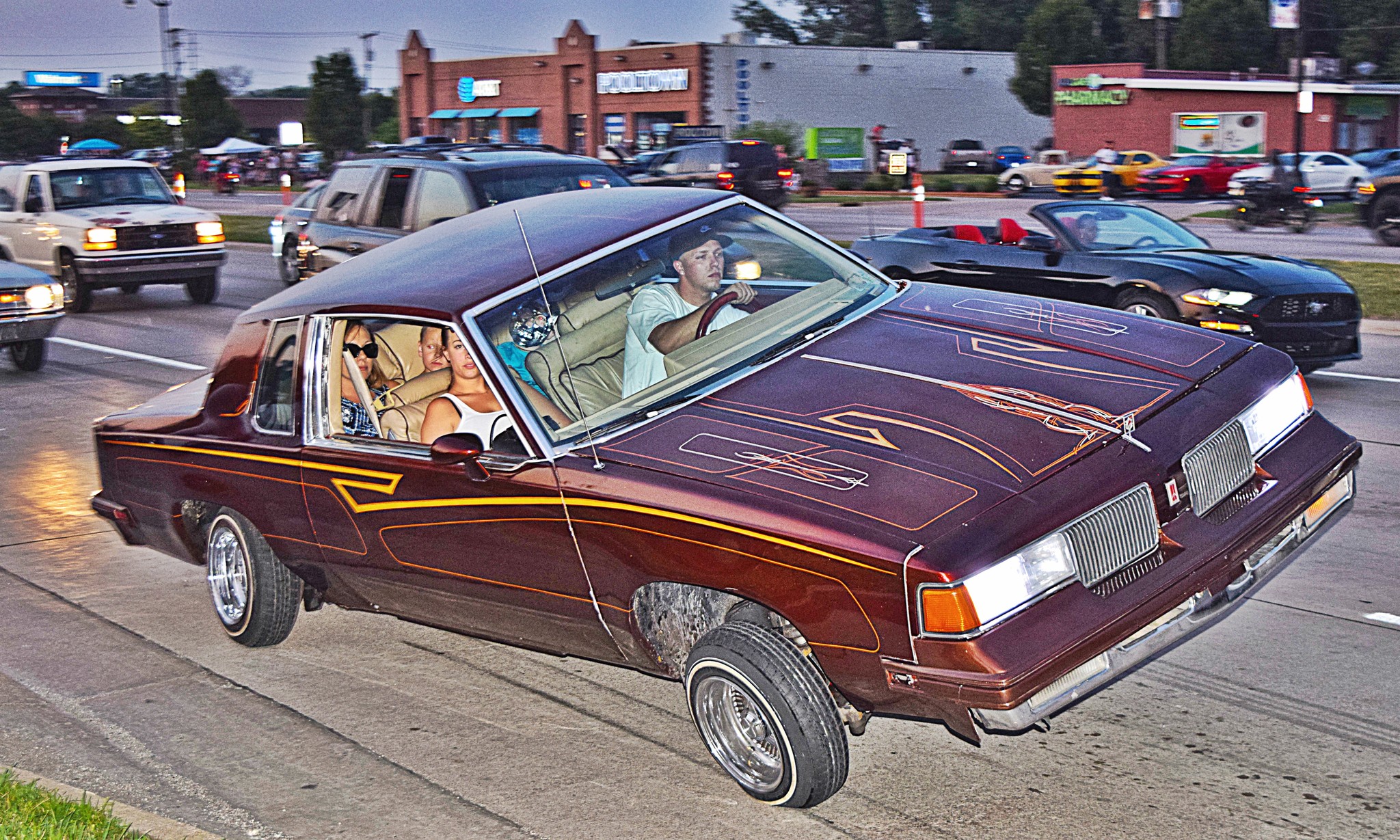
451;387;489;395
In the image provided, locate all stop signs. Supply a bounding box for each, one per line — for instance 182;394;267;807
60;141;69;154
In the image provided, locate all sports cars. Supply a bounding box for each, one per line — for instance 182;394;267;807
1134;151;1261;200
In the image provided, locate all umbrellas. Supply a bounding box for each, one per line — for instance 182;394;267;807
70;139;121;150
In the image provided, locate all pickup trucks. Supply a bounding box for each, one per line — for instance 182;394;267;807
846;199;1363;377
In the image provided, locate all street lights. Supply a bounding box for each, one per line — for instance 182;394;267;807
124;0;185;149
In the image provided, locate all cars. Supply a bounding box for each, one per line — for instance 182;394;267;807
876;138;921;175
995;147;1091;195
1;257;68;371
268;178;328;287
1049;149;1174;198
87;180;1366;813
1226;147;1400;248
115;134;665;188
993;145;1033;174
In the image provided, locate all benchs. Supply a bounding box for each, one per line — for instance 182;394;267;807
371;269;597;383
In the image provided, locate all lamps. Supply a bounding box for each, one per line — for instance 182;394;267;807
857;64;871;72
570;78;582;84
963;67;976;74
659;51;673;59
611;56;624;62
759;62;773;68
534;60;545;68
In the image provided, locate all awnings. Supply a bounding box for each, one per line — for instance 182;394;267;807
497;108;542;117
458;108;499;118
426;109;461;118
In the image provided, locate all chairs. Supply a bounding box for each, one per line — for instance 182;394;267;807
379;368;451;444
991;218;1029;246
524;297;632;422
947;223;987;243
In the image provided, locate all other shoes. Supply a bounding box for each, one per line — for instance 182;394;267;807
1099;196;1116;202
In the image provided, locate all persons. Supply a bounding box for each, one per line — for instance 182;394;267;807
417;326;452;375
1096;140;1118;201
106;173;135;197
1269;156;1285;182
494;293;561;399
1075;214;1098;245
774;144;787;157
51;185;72;205
197;150;296;182
341;319;402;437
621;224;758;400
421;328;574;451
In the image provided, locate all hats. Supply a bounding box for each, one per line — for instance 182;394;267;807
668;221;734;264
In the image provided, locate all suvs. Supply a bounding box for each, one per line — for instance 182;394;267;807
622;136;792;212
1;154;230;313
940;137;992;174
295;140;639;285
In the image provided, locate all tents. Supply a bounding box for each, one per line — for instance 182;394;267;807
201;138;277;155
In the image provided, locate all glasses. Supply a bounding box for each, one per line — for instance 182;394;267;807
342;343;379;358
